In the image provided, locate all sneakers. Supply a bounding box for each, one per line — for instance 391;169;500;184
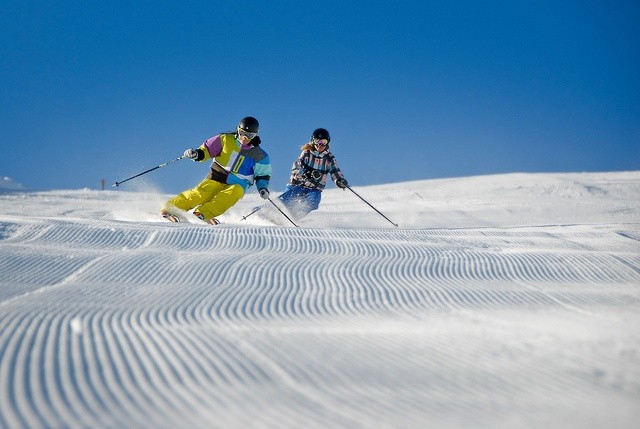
193;212;218;225
161;211;179;222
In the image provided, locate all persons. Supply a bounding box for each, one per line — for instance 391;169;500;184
281;128;347;221
163;115;272;223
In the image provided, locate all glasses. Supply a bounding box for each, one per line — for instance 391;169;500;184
238;127;257;140
312;138;329;146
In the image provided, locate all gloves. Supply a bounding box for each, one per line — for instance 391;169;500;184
260;189;268;198
335;178;348;188
311;170;322;180
184;149;199;160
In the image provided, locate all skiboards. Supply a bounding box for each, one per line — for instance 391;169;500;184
161;212;221;226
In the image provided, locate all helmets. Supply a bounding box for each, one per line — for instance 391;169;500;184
311;128;330;141
239;117;259;132
